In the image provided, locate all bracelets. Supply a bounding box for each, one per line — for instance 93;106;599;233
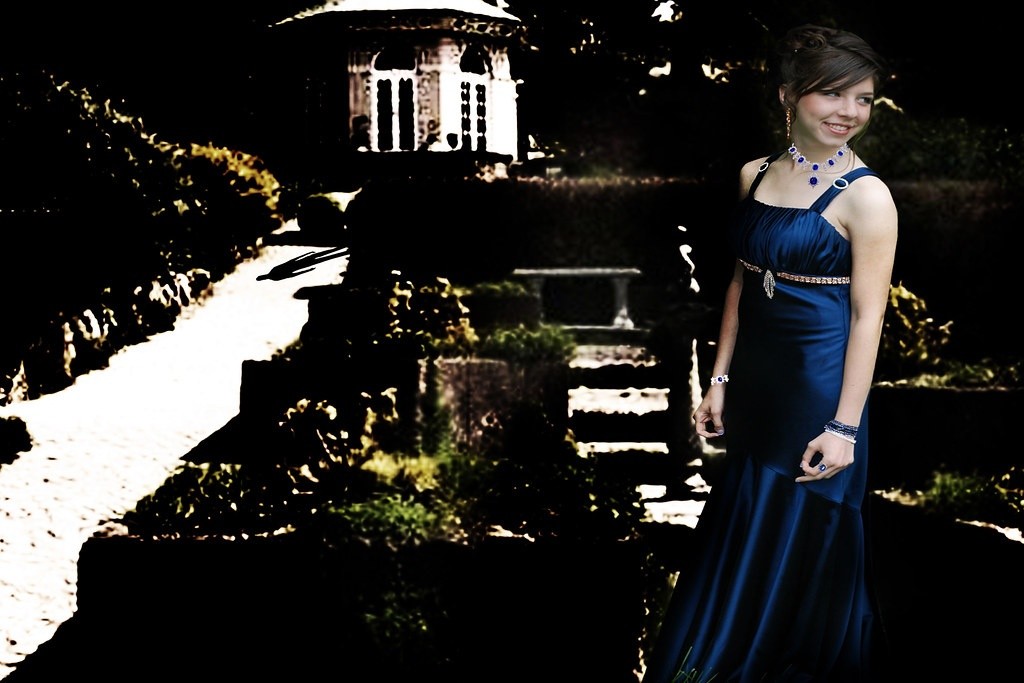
824;417;859;444
710;374;729;386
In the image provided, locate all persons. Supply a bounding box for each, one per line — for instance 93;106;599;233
666;25;902;683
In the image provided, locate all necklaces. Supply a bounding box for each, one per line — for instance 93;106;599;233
787;143;849;190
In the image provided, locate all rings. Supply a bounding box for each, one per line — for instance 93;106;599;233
817;461;827;472
